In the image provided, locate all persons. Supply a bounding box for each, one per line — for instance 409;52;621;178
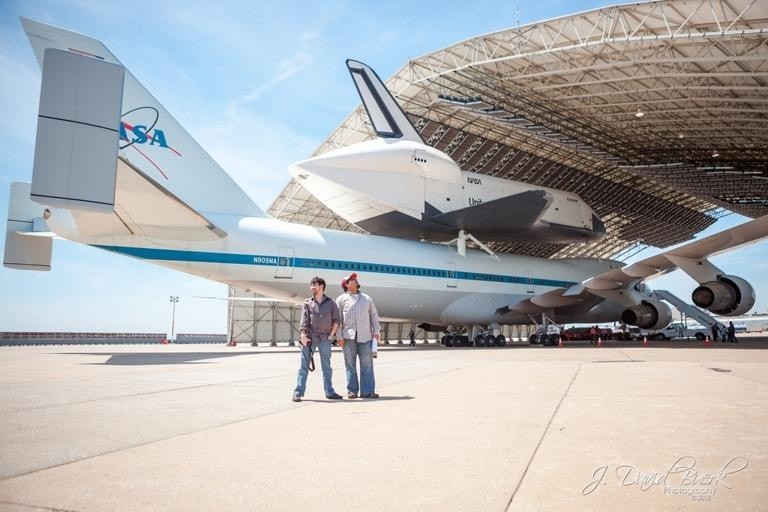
336;272;380;398
292;277;342;402
407;328;415;347
712;321;738;343
591;325;599;344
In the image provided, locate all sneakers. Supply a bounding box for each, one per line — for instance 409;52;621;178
348;391;379;398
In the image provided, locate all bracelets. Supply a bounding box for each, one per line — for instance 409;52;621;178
301;334;305;336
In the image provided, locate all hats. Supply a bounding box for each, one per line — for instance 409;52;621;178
341;272;357;289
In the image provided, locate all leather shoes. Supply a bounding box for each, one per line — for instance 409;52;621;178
292;394;301;402
326;393;342;399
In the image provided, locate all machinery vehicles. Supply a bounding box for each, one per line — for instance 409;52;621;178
653;289;729;342
